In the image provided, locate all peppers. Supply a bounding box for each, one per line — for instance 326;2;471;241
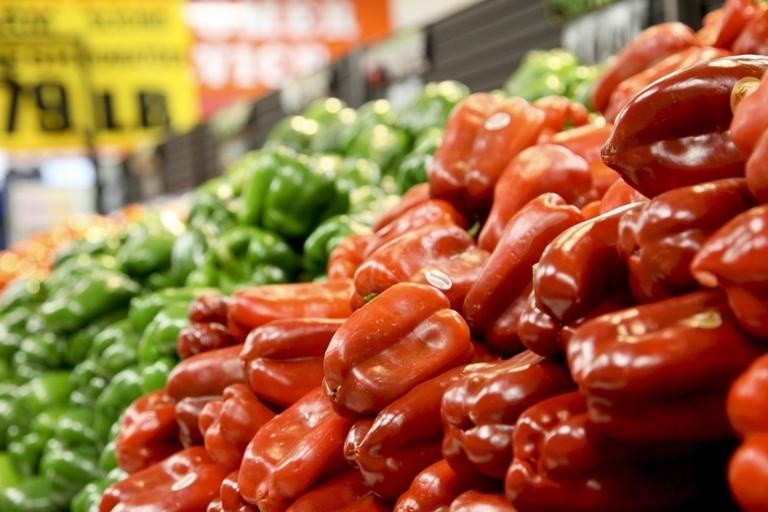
2;46;616;511
96;1;768;510
